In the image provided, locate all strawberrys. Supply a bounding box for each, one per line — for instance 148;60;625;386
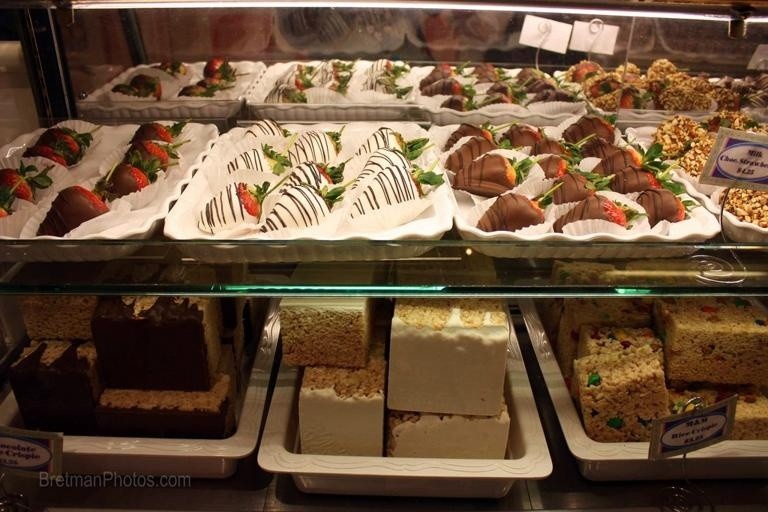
0;112;686;238
111;58;748;113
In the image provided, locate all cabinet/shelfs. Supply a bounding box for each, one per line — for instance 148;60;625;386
0;0;768;511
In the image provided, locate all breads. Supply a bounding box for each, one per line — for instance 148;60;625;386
8;247;510;460
